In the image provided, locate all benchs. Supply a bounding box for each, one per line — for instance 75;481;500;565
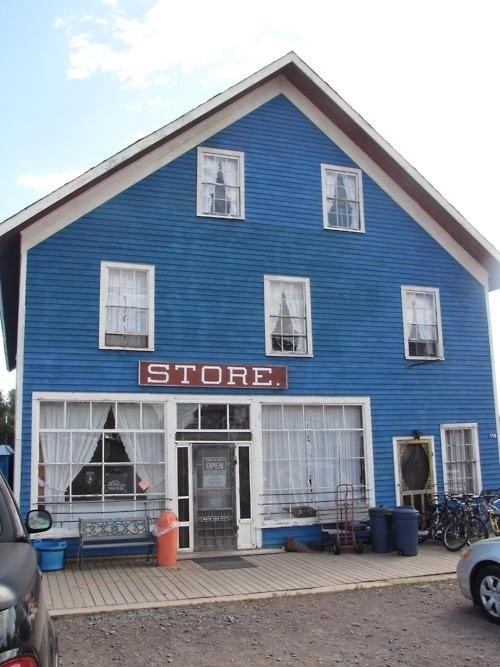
77;517;158;571
317;504;372;552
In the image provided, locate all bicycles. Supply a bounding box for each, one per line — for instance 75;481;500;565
419;489;500;552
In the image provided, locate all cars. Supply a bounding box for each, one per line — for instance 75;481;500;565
0;470;59;667
456;534;499;624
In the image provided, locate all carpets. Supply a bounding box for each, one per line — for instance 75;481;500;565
192;556;259;571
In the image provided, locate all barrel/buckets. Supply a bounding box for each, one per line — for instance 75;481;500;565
34;538;67;572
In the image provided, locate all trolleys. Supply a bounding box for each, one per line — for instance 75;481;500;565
332;484;363;554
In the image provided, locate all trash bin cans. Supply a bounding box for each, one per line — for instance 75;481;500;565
368;506;419;556
